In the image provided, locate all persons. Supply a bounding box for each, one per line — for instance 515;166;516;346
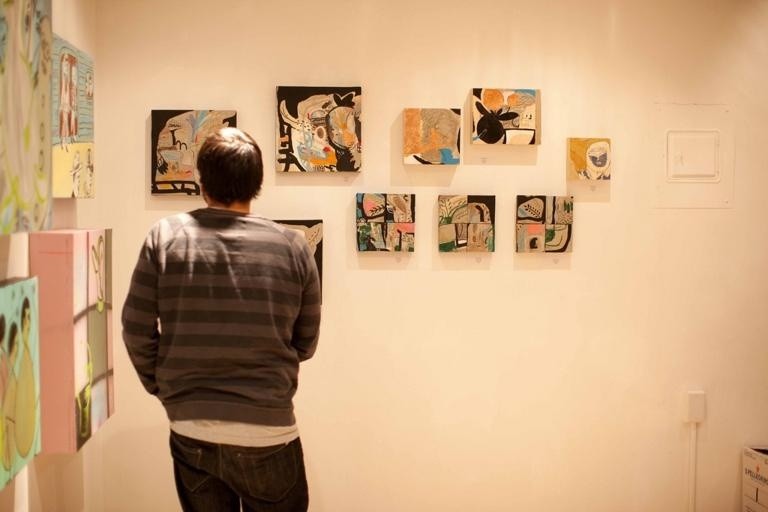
120;128;321;511
